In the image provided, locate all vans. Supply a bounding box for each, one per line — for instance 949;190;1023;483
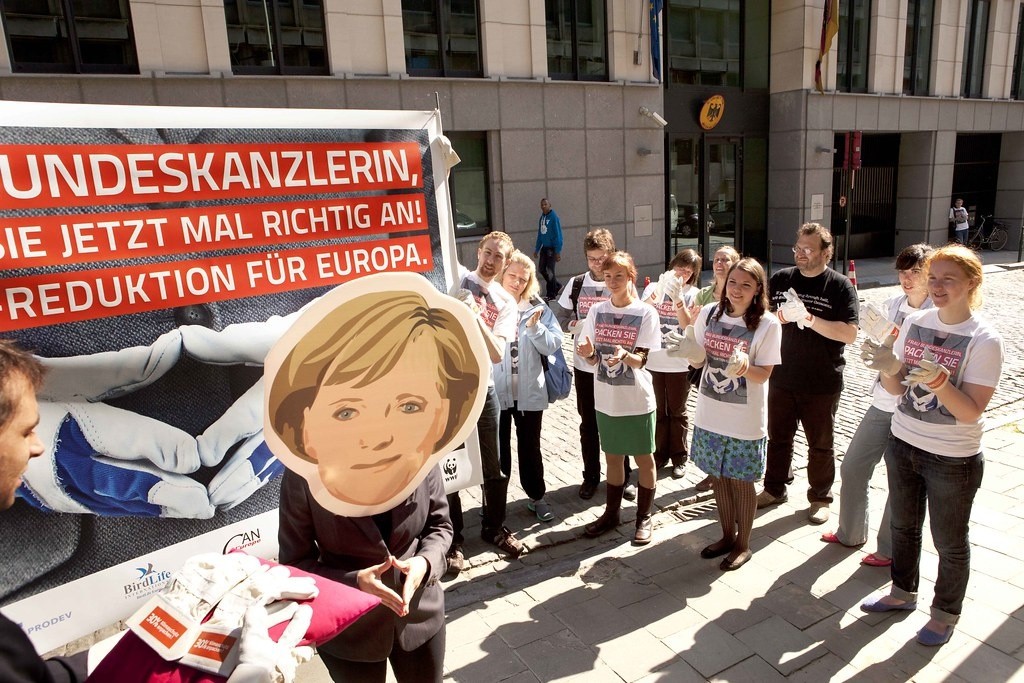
670;193;678;237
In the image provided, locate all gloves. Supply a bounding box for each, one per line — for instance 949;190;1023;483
125;552;323;682
455;270;685;317
858;300;951;393
664;325;707;363
776;287;816;331
719;342;750;380
8;309;289;523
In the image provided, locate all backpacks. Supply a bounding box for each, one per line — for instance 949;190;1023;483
539;312;573;403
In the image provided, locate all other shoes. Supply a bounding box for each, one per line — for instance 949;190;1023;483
860;599;917;613
719;548;752;571
701;541;736;558
862;553;891;566
916;625;955;646
822;531;859;546
579;458;832;525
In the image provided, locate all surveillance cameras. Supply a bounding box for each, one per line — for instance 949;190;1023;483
651;113;668;127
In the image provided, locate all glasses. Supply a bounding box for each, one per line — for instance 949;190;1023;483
585;255;607;263
792;245;822;255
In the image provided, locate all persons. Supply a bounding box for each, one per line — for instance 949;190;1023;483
533;198;564;302
860;243;1005;646
279;463;454;683
640;249;702;478
480;249;565;521
0;338;89;683
576;251;662;544
949;198;969;246
822;243;936;566
756;224;859;524
558;227;640;501
446;231;524;575
690;246;741;492
666;257;782;571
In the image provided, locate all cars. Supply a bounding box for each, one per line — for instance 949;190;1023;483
674;204;714;238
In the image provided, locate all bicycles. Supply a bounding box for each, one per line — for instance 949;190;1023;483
967;214;1008;251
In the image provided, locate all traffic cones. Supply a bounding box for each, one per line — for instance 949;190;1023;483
847;259;865;302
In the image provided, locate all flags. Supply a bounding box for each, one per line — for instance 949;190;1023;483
815;0;839;96
650;0;663;85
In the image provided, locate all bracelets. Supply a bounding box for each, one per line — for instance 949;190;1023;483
621;351;628;360
587;345;597;360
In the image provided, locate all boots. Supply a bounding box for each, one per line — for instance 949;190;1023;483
584;482;626;536
633;480;657;544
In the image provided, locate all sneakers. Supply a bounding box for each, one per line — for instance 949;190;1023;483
445;543;465;572
481;524;525;555
527;497;555;522
